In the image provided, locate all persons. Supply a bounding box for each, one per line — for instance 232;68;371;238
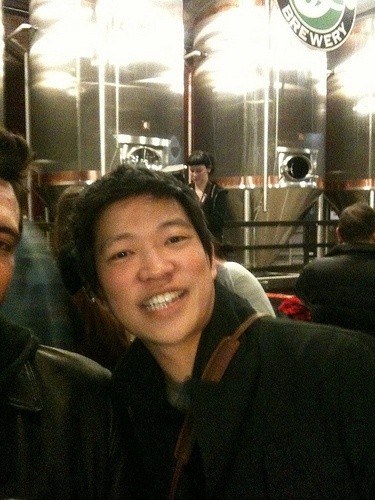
72;165;375;499
1;127;113;500
207;231;276;320
293;201;375;337
186;151;228;244
